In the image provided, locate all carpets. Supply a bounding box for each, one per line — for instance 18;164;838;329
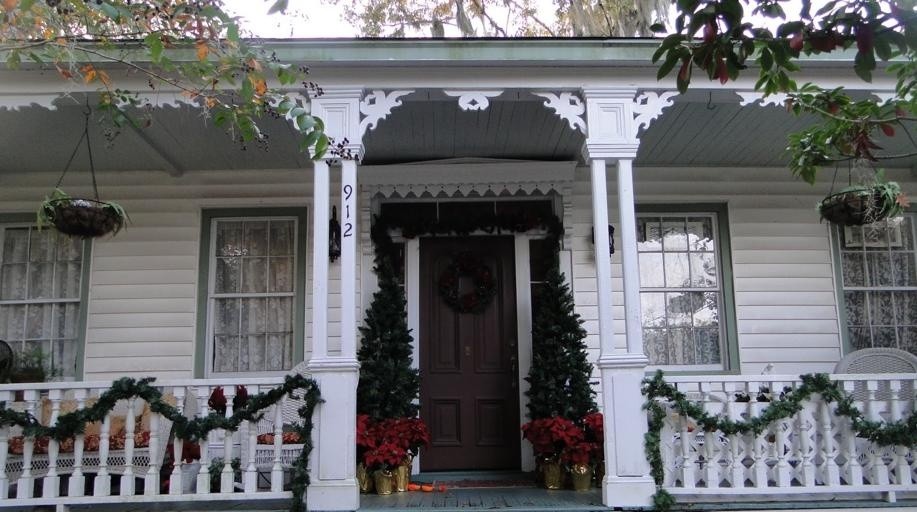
432;480;537;490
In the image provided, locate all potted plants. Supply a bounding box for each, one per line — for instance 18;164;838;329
815;169;912;226
7;344;54;383
36;187;133;246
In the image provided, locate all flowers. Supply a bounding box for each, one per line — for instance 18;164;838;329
522;413;603;464
438;253;497;314
357;413;432;477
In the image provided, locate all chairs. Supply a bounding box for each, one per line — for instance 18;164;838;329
831;347;917;401
254;359;313;490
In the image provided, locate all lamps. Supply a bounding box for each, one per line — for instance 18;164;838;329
329;206;341;262
592;224;614;258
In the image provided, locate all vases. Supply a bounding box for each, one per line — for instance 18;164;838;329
357;461;412;495
543;463;605;491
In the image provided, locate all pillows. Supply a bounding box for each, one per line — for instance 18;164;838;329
8;393;177;454
256;432;301;444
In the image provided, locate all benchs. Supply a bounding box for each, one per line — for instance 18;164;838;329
8;394;175;486
